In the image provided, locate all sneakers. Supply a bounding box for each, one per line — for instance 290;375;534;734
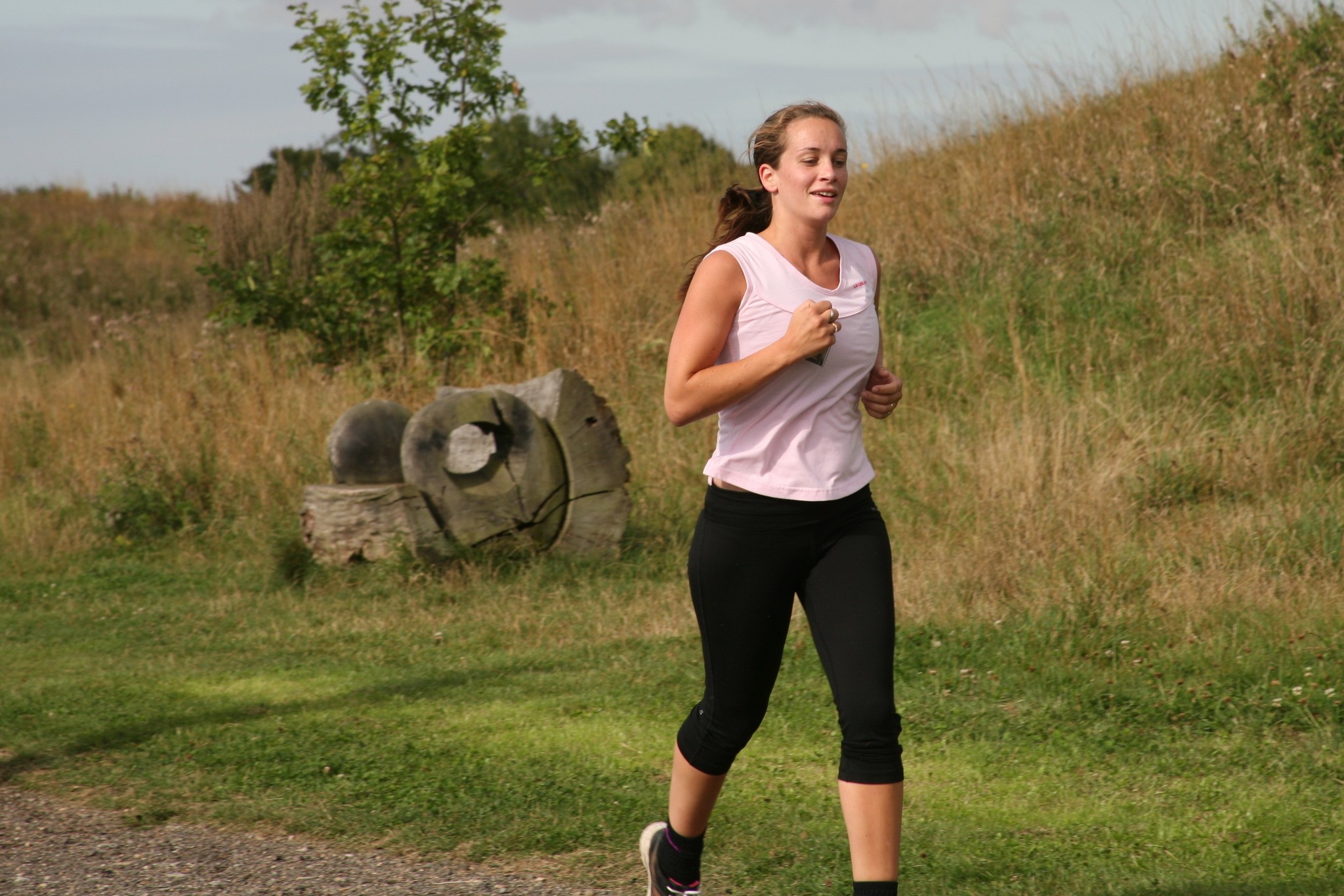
639;820;702;896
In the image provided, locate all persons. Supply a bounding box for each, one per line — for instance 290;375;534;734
637;103;908;896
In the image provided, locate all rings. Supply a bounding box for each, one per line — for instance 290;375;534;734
887;404;893;414
832;323;838;333
829;307;833;322
